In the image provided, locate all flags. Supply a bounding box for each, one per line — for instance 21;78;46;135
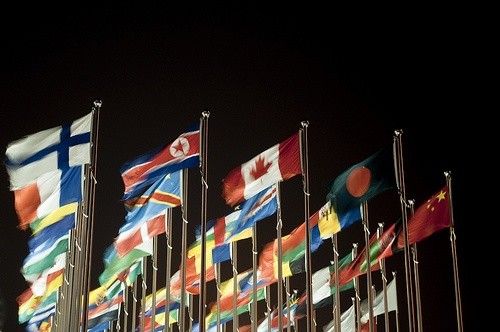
337;231;382;286
322;295;375;332
4;99;320;332
328;153;389;213
396;184;453;249
358;222;398;273
311;250;353;304
359;276;396;324
318;196;365;240
